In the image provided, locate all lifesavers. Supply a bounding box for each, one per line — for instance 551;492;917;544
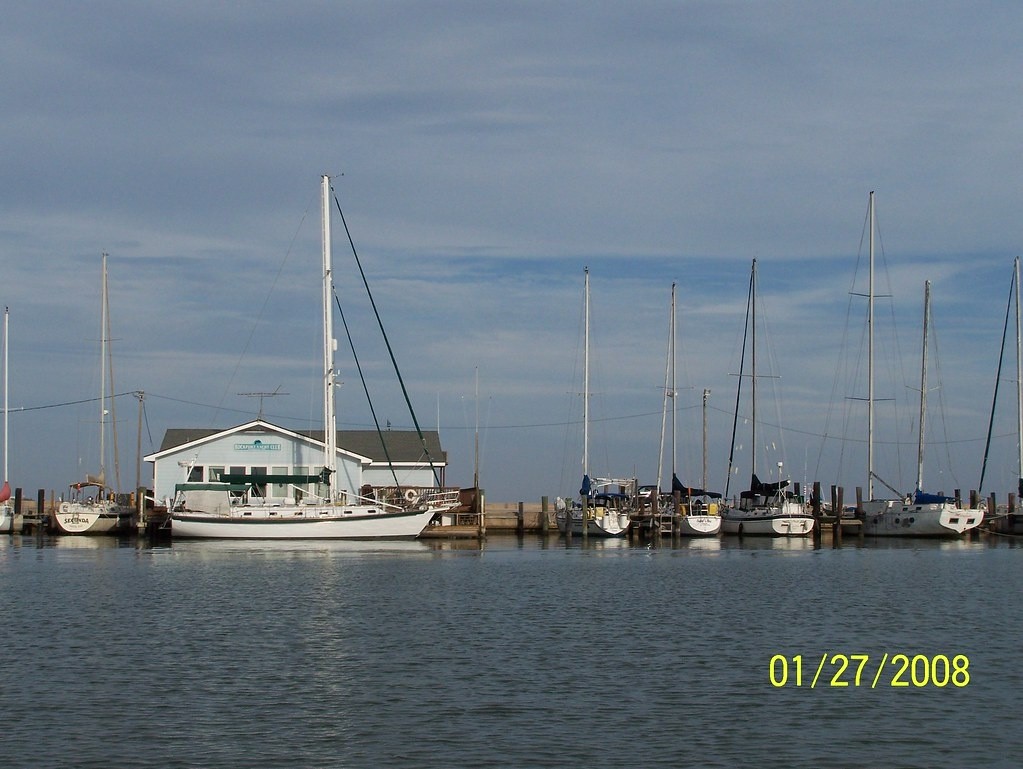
680;506;686;516
405;489;417;502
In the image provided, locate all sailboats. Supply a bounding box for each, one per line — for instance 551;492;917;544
862;279;987;538
0;302;25;533
720;259;818;537
46;250;125;534
151;168;463;541
549;265;634;535
634;282;725;537
981;255;1023;535
808;189;906;539
